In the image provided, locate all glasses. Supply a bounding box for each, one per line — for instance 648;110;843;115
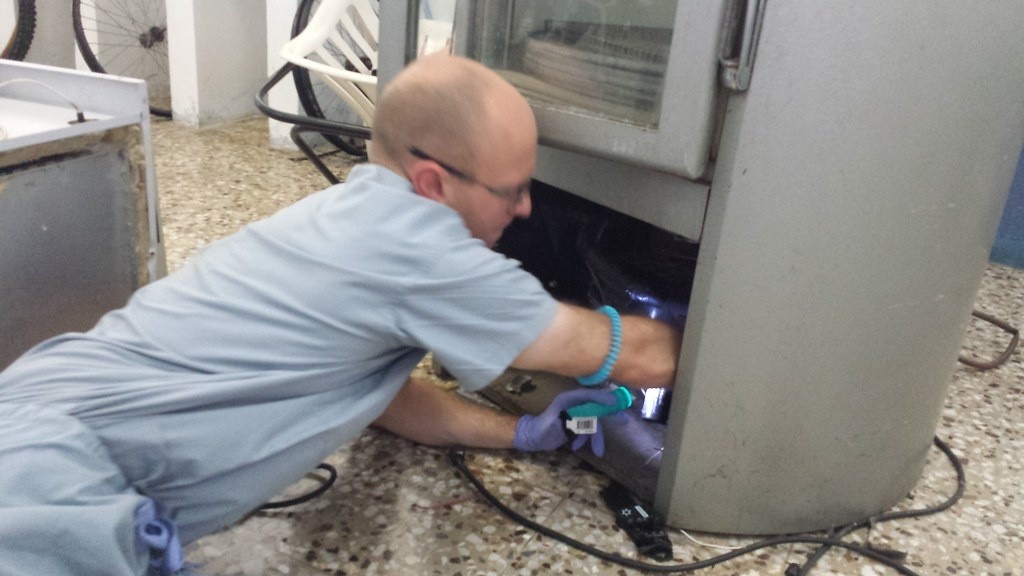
409;141;536;204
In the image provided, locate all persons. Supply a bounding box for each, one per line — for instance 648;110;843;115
0;57;682;576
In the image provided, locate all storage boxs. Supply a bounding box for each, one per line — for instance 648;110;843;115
279;0;453;127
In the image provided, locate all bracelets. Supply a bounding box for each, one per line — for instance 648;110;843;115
578;306;622;384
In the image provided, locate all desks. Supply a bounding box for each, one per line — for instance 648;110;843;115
0;58;170;375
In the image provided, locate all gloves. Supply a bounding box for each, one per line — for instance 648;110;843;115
513;387;619;456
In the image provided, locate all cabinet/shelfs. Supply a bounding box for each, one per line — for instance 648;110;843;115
377;0;1024;538
